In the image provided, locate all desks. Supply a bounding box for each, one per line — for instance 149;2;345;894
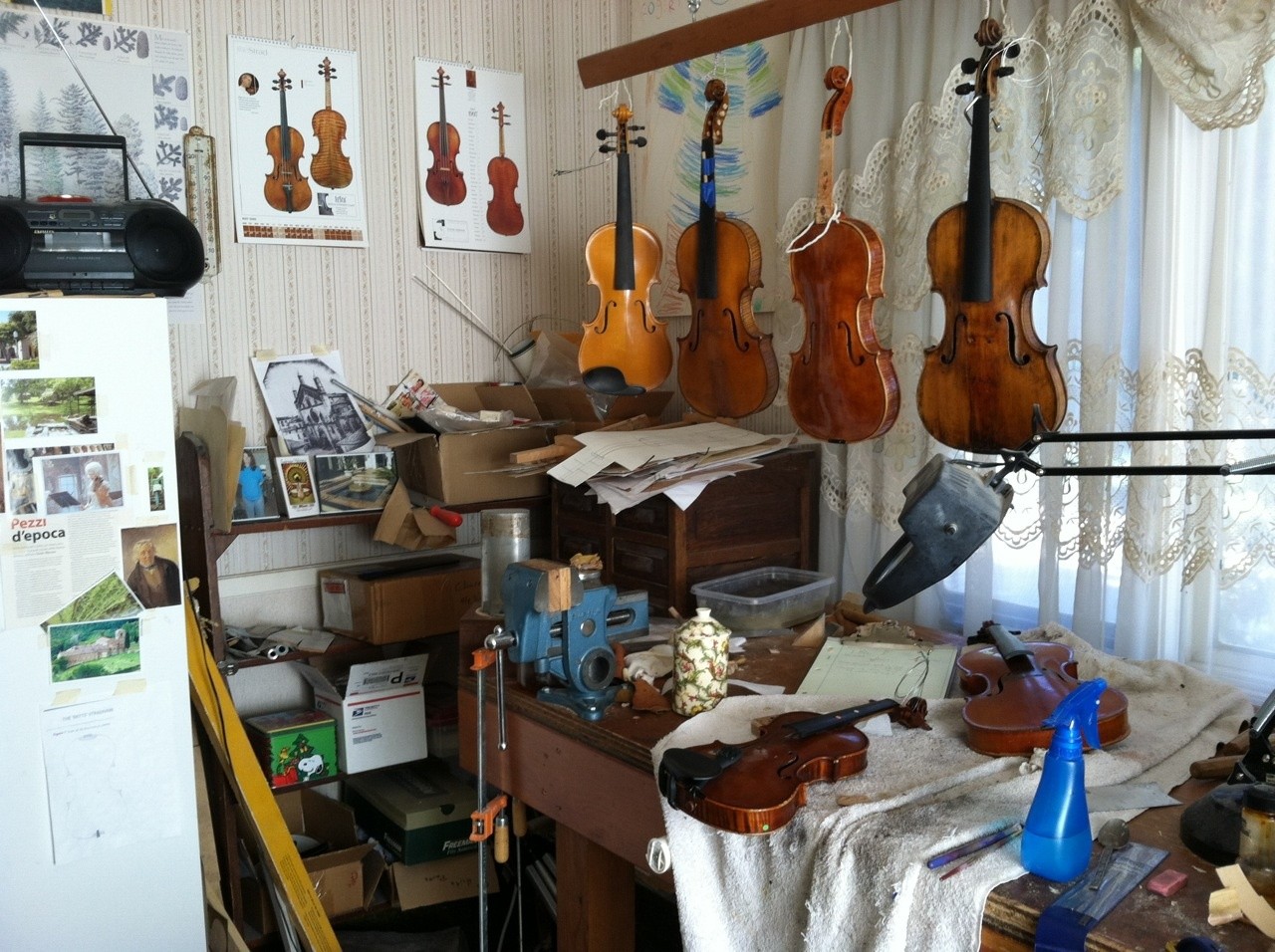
459;618;1275;950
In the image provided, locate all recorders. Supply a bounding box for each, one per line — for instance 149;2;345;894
0;0;205;297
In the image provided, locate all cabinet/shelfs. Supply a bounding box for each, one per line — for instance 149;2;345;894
174;431;550;951
551;443;821;619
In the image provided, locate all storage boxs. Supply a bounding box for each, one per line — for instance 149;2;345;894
690;566;835;633
233;760;500;937
423;680;458;759
317;553;482;645
376;383;676;506
243;708;338;788
296;652;430;774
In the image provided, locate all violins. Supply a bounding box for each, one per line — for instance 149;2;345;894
914;15;1068;453
674;78;780;419
424;66;467;206
957;621;1132;757
576;101;673;396
787;65;901;446
264;69;312;214
310;56;353;190
486;101;524;236
658;696;933;836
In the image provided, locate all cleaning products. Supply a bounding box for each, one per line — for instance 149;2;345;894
1015;678;1111;886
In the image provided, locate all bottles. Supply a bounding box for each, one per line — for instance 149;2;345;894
673;606;730;716
1238;784;1275;868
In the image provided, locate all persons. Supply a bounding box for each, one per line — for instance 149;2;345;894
125;538;182;608
83;461;114;510
391;395;414;417
289;477;311;502
150;470;163;511
238;451;268;517
408;378;425;396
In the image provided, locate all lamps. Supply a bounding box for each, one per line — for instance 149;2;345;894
861;402;1275;614
